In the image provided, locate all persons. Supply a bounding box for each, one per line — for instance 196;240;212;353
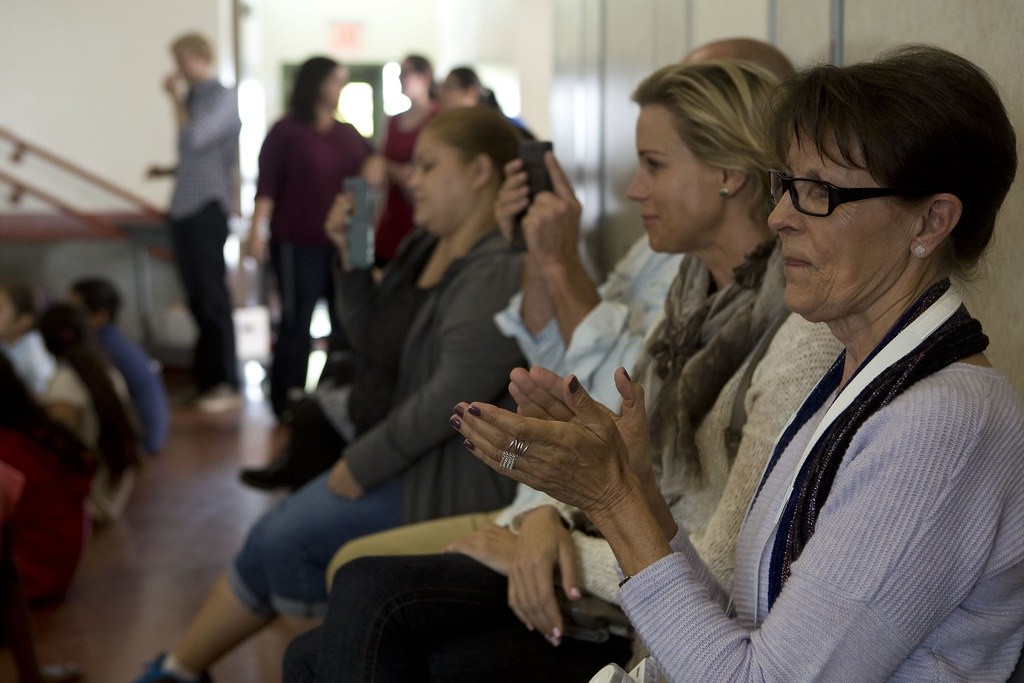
0;270;169;683
135;35;1024;683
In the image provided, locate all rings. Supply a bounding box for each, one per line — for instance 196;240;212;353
508;437;530;455
499;451;517;469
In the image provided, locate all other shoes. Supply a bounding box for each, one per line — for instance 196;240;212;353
127;653;218;683
235;454;304;497
200;384;248;415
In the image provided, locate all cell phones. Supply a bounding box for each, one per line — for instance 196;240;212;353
521;140;554;205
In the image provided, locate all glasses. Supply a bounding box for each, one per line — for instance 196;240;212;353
766;168;924;218
